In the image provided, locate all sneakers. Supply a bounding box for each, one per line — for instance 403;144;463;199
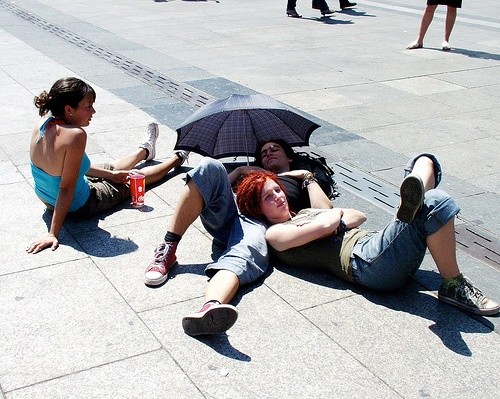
143;241;178;285
181;303;239;336
138;121;159;161
437;276;500;315
174;149;190;165
396;174;426;223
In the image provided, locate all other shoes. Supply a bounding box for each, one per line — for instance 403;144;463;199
441;40;450;50
406;42;424;49
339;2;357;10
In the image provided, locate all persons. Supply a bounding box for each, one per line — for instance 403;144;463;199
143;139;334;336
339;0;357;9
406;0;462;51
26;77;190;254
236;154;500;316
286;0;335;18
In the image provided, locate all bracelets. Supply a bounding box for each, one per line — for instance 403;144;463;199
302;172;317;187
340;220;350;231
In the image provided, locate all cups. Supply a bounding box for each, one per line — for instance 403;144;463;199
129;175;146;209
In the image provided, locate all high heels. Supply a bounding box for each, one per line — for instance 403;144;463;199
320;10;335;17
287;10;302;18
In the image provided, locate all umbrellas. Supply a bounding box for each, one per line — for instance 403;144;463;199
174;94;321;167
